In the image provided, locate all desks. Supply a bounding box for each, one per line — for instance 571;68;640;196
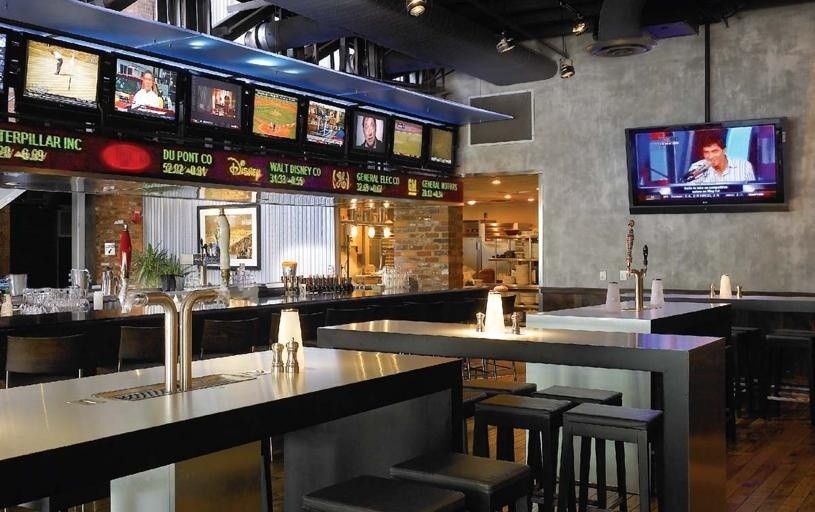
316;321;729;512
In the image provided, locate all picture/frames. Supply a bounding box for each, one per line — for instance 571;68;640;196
195;205;261;270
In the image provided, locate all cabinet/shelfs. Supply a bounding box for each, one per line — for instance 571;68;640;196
488;234;539;288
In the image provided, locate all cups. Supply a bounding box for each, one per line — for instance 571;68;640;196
229;264;255;287
21;284;89;320
144;302;181;315
191;299;226;311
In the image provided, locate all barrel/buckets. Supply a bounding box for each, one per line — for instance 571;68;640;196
7;274;27;297
514;264;529;286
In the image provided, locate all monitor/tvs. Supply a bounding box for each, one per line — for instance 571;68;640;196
392;114;427;168
303;94;348;157
247;83;304;152
624;117;789;214
15;31;110;116
0;27;14;112
107;51;181;132
348;107;390;160
183;68;248;141
428;123;456;169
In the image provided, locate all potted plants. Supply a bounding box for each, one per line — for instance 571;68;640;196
132;241;192;292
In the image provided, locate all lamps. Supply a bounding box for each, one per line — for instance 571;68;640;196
404;1;586;79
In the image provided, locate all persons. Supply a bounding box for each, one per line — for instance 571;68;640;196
273;123;276;129
224;91;231;114
132;70;164;110
685;135;756;182
361;117;381;148
46;49;64;74
270;120;272;129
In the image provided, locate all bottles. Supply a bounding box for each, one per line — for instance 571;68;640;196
720;275;732;297
382;265;411;295
651;280;665;307
476;312;485;332
270;341;284;367
284;338;298;374
510;311;521;334
710;283;716;296
172;294;178;302
279;309;303;357
606;281;622;313
737;285;743;297
284;274;367;296
483;292;505;333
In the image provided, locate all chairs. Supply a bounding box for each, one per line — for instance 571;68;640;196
400;300;445;321
325;304;383;327
442;294;517;383
199;316;257;356
6;333;86;389
271;311;324;349
116;325;179;371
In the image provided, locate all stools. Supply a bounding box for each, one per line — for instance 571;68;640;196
475;395;570;508
534;385;629;509
461;379;491;512
470;372;541;512
558;402;662;512
